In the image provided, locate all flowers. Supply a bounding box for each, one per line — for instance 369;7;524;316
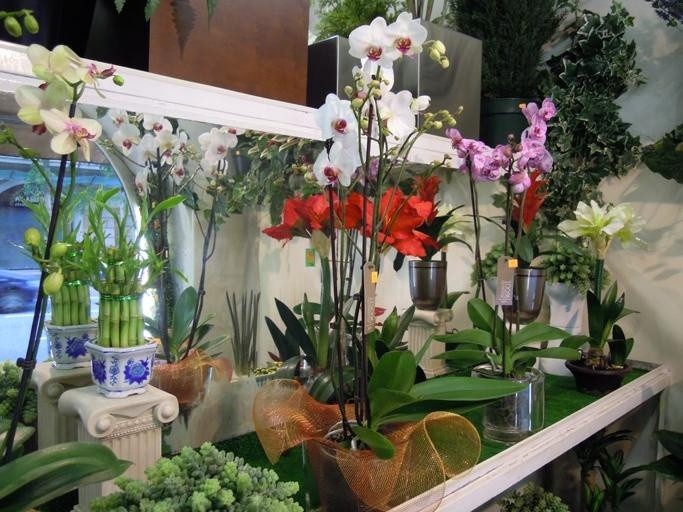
390;176;475;272
555;201;648;368
80;102;246;368
0;44;131;511
259;173;444;404
311;12;469;442
449;96;598;379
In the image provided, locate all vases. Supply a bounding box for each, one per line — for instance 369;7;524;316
148;350;209;413
498;269;546;325
306;426;415;511
472;363;546;446
411;260;444;310
564;356;630;396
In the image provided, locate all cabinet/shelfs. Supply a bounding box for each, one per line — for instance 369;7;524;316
0;31;671;511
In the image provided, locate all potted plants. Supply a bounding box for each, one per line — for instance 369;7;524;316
81;196;184;400
22;184;98;371
